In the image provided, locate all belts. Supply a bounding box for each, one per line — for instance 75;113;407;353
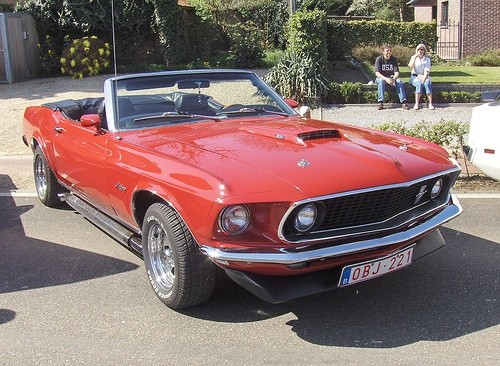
412;73;417;77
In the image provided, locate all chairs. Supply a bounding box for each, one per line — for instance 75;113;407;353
172;92;208;116
97;97;134;130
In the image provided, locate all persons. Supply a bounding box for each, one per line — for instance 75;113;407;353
408;44;434;110
374;46;409;110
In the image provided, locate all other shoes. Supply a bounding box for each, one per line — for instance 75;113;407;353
377;103;383;109
414;103;420;110
402;103;410;110
429;105;434;110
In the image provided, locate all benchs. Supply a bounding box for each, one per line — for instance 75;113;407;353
41;94;176;120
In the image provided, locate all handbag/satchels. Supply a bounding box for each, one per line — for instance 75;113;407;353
417;74;427;80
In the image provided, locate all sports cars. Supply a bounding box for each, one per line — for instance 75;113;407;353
21;68;464;311
